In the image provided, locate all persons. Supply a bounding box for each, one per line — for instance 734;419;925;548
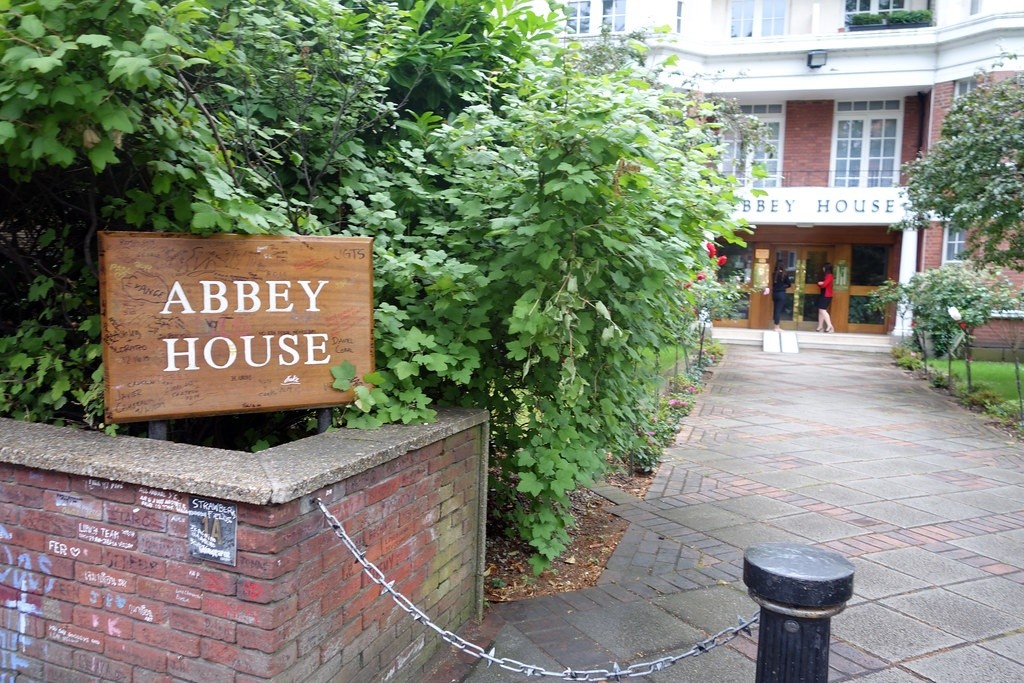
815;263;834;333
772;260;792;332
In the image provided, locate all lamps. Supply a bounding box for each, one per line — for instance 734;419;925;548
808;50;828;68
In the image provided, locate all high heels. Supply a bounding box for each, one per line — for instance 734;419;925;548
815;327;824;333
825;325;834;333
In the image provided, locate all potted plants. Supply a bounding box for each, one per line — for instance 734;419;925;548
849;10;934;31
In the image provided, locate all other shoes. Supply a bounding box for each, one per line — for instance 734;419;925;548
774;328;784;332
